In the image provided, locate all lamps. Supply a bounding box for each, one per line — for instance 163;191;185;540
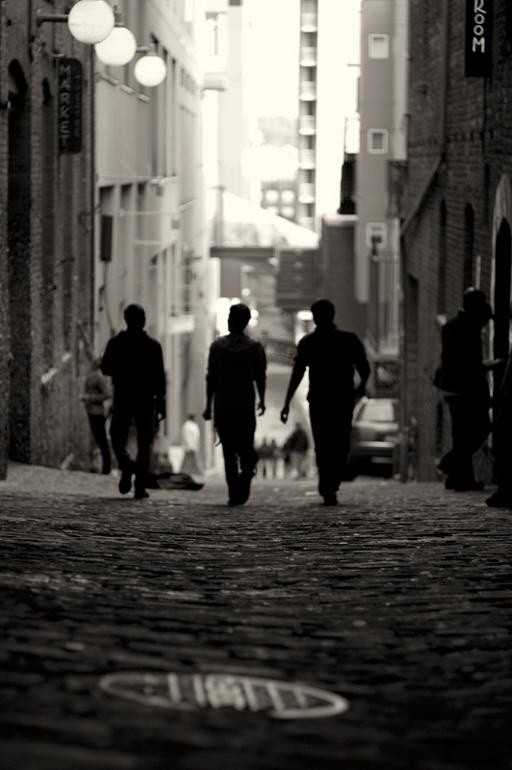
36;0;168;88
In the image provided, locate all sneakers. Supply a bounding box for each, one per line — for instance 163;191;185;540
119;464;338;507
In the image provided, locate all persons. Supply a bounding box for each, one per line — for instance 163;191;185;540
81;329;111;475
180;413;203;476
255;422;309;480
279;297;371;507
98;303;167;499
200;302;268;508
432;286;492;493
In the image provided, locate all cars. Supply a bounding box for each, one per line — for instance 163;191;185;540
344;395;399;482
